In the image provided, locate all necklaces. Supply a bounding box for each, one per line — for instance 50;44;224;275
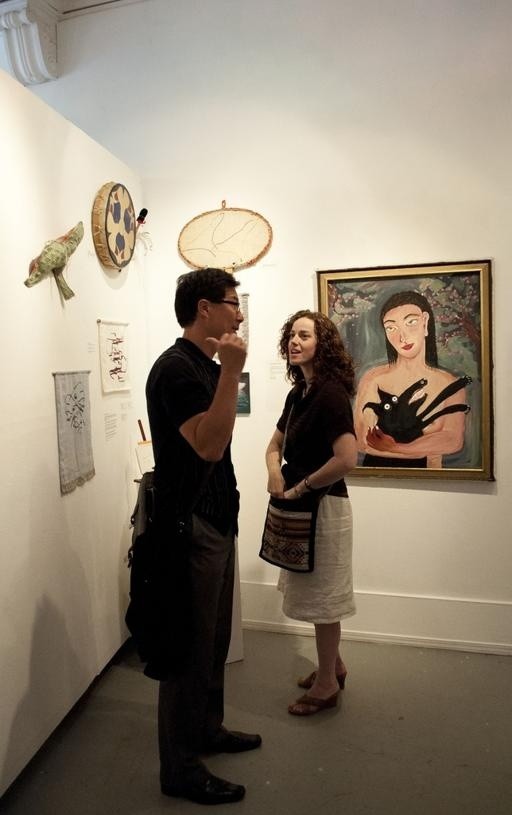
303;385;307;397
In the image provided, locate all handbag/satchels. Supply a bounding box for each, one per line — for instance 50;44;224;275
259;494;320;573
128;471;185;570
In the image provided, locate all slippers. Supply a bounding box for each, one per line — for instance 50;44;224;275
287;689;340;716
298;670;347;690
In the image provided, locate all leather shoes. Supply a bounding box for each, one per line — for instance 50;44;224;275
161;772;245;805
215;731;262;753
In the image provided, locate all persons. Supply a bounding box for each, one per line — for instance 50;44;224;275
140;268;264;806
266;308;361;718
351;291;468;469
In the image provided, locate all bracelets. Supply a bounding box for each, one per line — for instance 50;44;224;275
294;483;303;498
305;475;316;492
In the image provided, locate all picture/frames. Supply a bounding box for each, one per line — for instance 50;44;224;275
315;259;494;482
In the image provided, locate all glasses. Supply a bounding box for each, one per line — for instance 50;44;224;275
219;299;240;313
